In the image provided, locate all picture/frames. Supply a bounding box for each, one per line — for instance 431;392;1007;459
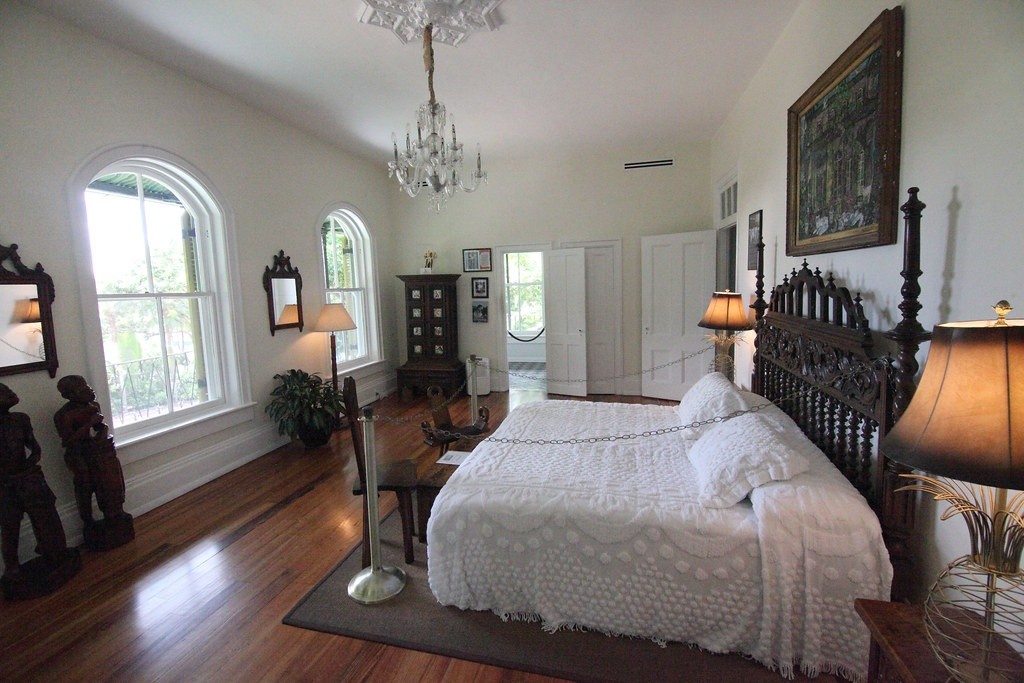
785;5;904;256
747;209;762;270
471;277;489;299
462;248;492;272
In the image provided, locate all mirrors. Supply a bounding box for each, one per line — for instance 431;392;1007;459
262;249;305;336
0;242;59;379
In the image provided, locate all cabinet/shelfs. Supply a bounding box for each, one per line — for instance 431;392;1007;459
395;273;466;399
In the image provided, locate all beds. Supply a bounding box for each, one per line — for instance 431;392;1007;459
426;186;927;683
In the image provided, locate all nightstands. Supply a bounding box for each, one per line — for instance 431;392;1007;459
852;598;1024;683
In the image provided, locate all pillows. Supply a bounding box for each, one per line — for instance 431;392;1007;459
673;371;811;508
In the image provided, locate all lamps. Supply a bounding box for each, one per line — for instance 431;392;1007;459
696;289;753;384
278;304;300;324
876;300;1024;683
21;298;45;360
313;303;357;431
361;0;510;213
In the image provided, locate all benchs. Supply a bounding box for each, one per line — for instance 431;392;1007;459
418;436;481;543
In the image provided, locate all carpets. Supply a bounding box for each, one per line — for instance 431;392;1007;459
282;489;843;683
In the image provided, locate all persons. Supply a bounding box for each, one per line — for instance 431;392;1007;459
54;375;129;528
0;385;68;581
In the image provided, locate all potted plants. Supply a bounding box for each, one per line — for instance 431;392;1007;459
264;369;348;447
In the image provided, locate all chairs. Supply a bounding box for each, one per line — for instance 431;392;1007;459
421;385;490;458
341;375;418;570
